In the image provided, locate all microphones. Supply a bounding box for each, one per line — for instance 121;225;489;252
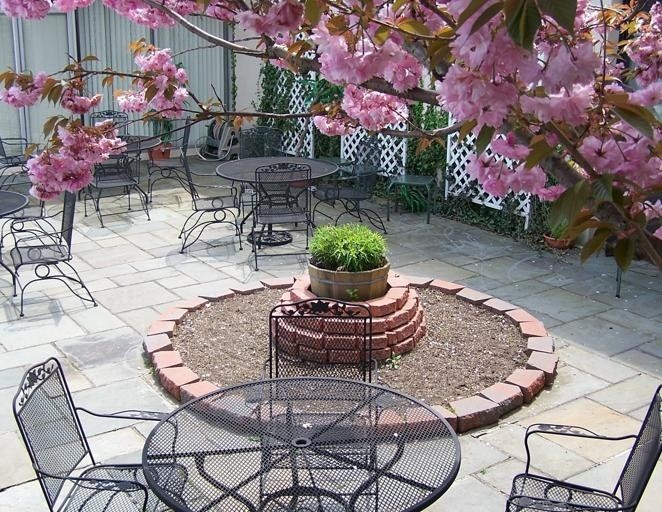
386;174;437;224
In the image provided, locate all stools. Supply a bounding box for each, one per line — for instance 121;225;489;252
386;174;437;224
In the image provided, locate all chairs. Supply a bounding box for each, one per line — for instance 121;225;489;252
261;297;378;510
177;126;387;271
13;357;171;511
505;386;662;512
78;109;200;228
0;134;98;317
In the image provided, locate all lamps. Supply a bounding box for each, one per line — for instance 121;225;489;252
177;126;387;271
78;109;200;228
0;134;98;317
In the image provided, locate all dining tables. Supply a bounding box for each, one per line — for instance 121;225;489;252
309;222;389;300
543;220;576;249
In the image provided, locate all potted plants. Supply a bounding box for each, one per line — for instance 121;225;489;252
309;222;389;300
543;220;576;249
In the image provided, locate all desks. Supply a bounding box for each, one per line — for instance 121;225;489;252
142;377;461;512
